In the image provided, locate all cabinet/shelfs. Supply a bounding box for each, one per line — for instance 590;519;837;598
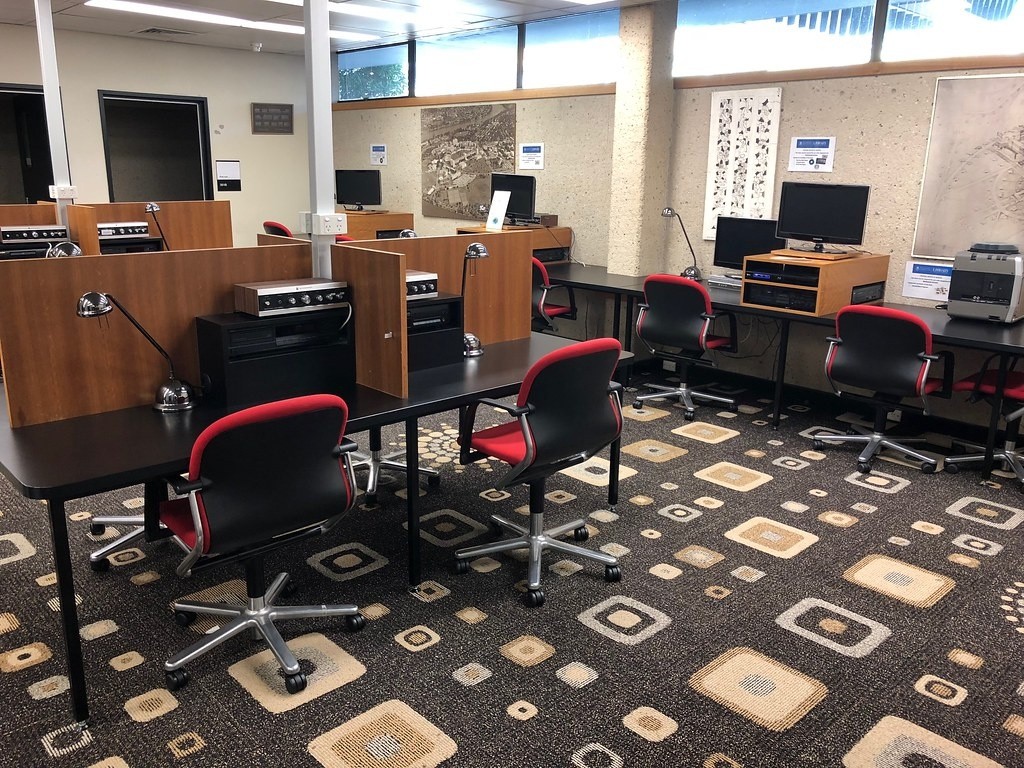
190;305;357;418
734;253;892;316
335;211;415;240
406;291;465;372
453;227;573;264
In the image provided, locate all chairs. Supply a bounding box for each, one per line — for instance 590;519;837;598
156;392;364;696
336;235;354;242
531;256;579;334
448;335;626;609
264;221;294;238
87;510;193;573
631;273;740;420
341;424;441;507
812;304;954;475
942;352;1024;495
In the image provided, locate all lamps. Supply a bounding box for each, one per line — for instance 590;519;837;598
661;207;703;281
461;241;490;359
145;202;171;252
75;291;198;411
42;240;83;258
398;228;418;238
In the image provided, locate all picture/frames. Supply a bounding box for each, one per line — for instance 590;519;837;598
911;73;1024;263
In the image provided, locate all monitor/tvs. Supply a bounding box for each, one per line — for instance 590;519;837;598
713;217;786;279
335;170;382;211
775;181;871;254
490;172;536;226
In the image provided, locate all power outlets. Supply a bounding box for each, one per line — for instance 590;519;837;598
312;213;347;234
56;186;78;199
299;211;312;234
49;185;56;198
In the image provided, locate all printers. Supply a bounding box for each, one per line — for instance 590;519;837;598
946;252;1024;323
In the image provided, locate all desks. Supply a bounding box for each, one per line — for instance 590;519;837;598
0;234;1024;721
